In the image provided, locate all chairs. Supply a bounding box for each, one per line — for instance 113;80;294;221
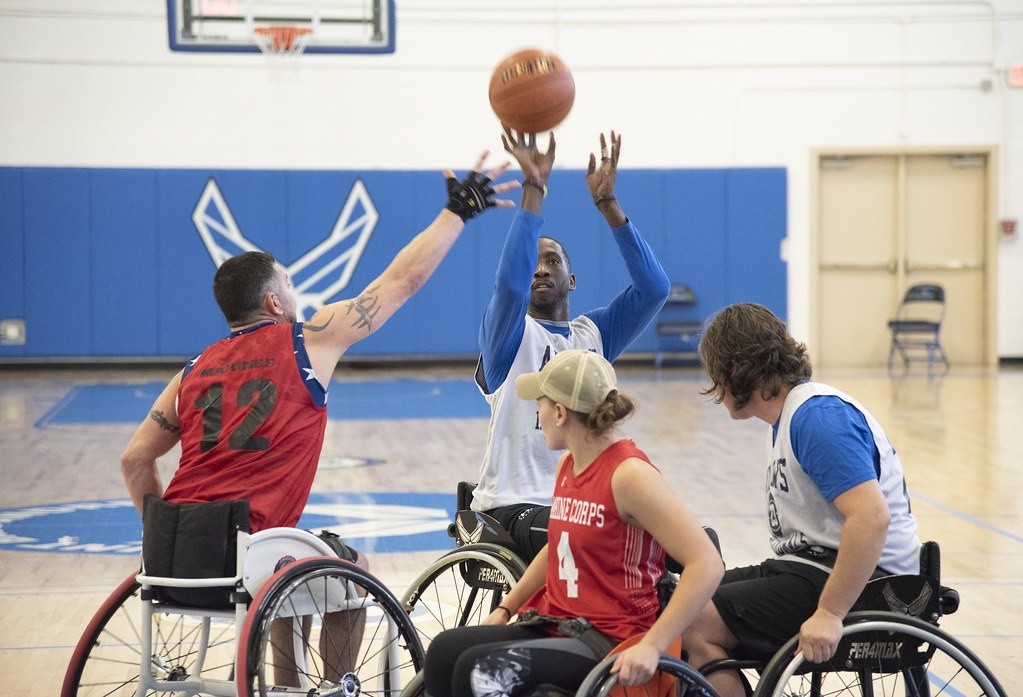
888;284;951;374
655;283;703;369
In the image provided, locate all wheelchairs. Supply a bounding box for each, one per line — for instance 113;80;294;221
399;570;719;697
377;481;528;697
685;540;1007;697
60;493;426;697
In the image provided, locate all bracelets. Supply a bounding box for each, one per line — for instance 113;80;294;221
522;178;547;198
495;606;511;620
594;197;616;205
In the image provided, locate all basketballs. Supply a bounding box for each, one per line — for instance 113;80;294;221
488;47;576;135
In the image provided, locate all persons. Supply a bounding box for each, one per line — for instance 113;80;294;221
120;150;520;697
423;349;724;697
473;129;719;608
682;304;921;697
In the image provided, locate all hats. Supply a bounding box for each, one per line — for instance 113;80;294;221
516;349;618;414
